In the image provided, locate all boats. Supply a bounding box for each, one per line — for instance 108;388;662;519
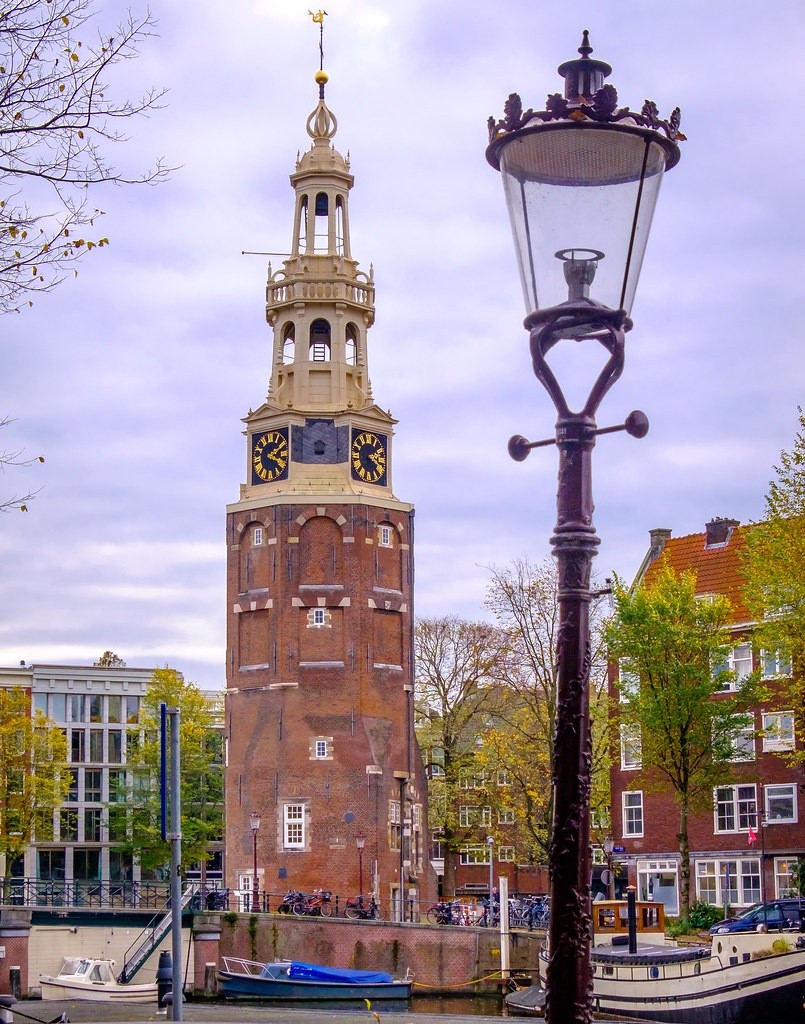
505;890;805;1024
39;956;187;1004
217;954;415;1002
0;994;70;1024
538;898;679;990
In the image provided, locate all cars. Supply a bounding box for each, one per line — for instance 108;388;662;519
707;899;805;937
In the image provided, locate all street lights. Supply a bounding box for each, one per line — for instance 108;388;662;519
759;810;771;901
485;31;687;1024
355;830;366;908
602;834;616;898
487;836;496;928
249;811;262;913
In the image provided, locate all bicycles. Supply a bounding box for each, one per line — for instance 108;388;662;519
346;892;386;921
426;894;551;931
293;891;333;917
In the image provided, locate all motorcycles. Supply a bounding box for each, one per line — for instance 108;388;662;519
278;890;320;916
188;886;227;911
